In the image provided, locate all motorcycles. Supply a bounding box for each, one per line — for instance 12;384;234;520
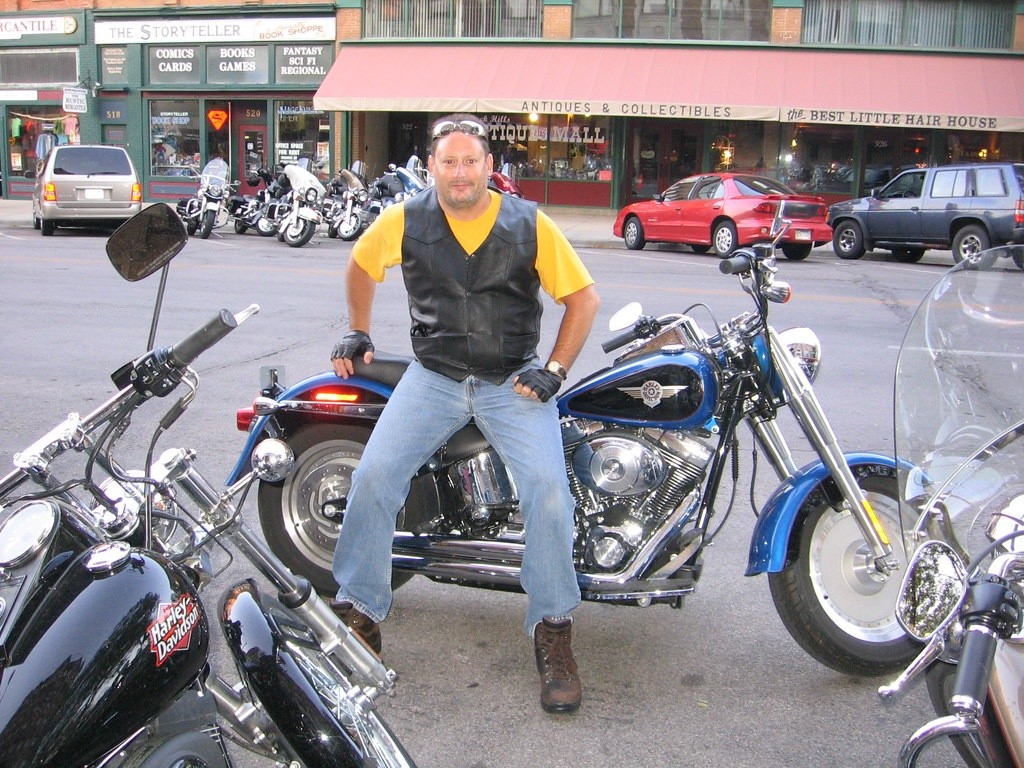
1;200;422;768
176;155;529;253
879;241;1024;768
222;199;963;679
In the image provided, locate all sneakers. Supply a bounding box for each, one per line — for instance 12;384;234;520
534;622;581;714
326;598;381;677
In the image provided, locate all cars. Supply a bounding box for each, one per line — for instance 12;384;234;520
613;170;835;263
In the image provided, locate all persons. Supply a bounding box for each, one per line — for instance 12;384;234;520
329;113;601;713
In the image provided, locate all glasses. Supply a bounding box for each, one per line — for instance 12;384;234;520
432;120;488;143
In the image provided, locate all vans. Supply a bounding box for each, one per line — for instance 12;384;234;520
31;141;144;237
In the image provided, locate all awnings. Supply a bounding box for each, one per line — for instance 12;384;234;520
313;42;1024;134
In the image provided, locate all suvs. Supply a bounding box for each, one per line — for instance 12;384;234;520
827;160;1023;276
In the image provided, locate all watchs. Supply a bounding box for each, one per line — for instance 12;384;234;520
545;360;567;380
